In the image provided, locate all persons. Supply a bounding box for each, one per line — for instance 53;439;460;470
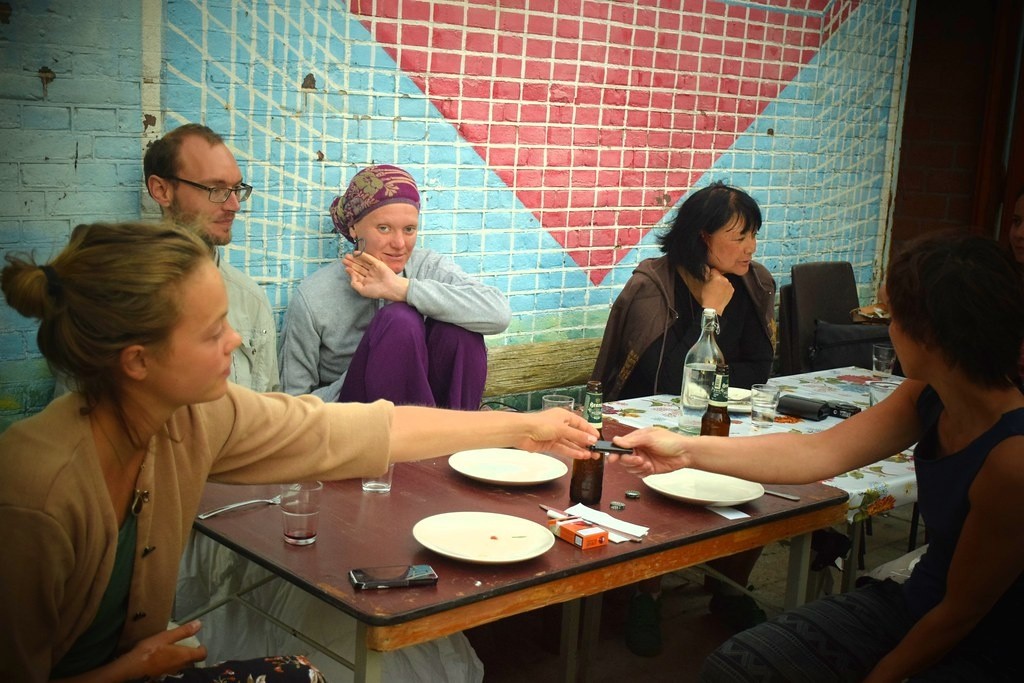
1006;186;1024;263
0;222;601;683
278;165;512;411
607;226;1024;683
143;123;484;683
588;179;774;657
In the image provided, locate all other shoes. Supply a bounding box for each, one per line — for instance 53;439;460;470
626;601;664;656
709;590;767;632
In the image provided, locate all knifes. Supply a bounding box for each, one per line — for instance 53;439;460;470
539;503;598;526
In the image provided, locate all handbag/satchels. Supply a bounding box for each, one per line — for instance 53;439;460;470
806;318;904;377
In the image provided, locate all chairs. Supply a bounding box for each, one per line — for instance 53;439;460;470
779;260;860;377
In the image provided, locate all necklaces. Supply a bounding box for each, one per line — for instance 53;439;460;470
86;402;149;516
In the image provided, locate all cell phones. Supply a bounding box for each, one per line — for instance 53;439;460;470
349;564;439;590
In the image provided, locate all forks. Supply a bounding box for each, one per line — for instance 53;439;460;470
198;484;302;519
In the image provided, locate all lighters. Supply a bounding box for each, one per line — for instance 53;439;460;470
589;441;633;454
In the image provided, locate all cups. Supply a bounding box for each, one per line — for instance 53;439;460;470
279;482;323;546
750;383;780;429
542;394;575;412
361;462;395;493
869;381;900;407
872;343;897;379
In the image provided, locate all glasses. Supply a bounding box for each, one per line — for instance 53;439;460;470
155;173;253;204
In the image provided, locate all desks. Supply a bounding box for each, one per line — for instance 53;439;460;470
765;365;920;451
175;408;850;683
589;388;917;609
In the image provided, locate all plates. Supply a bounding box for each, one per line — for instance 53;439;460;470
722;386;779;413
448;448;569;486
412;511;556;565
642;468;765;507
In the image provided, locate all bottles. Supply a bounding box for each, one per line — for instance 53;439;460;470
677;308;726;435
569;380;606;506
699;363;732;437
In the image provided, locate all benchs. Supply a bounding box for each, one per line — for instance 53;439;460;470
481;320;778;398
